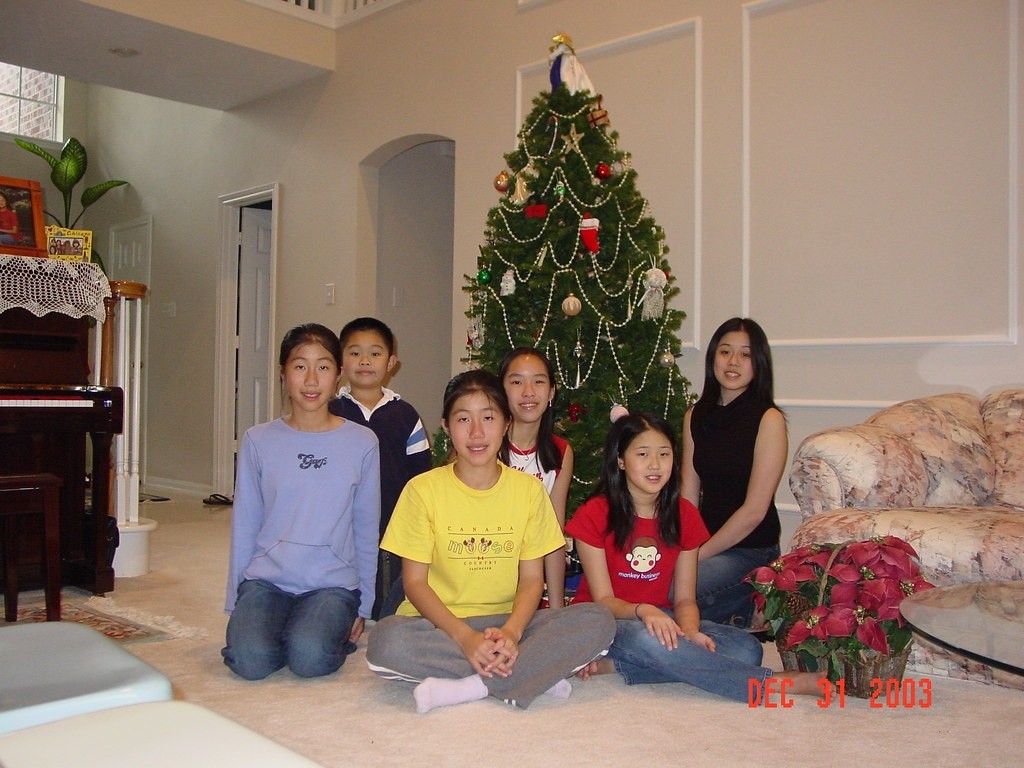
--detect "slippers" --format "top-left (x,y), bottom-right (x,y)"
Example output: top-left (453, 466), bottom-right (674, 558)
top-left (203, 493), bottom-right (234, 505)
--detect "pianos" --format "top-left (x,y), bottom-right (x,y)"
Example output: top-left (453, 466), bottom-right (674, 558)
top-left (0, 254), bottom-right (124, 598)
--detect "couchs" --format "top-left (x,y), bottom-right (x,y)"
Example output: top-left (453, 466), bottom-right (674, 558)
top-left (786, 388), bottom-right (1024, 691)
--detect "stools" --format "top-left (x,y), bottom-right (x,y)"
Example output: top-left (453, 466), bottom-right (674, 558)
top-left (0, 472), bottom-right (64, 625)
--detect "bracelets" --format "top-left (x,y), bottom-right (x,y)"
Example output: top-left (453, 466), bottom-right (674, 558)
top-left (634, 600), bottom-right (643, 622)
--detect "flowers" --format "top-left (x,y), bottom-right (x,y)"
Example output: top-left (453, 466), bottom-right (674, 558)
top-left (740, 536), bottom-right (937, 683)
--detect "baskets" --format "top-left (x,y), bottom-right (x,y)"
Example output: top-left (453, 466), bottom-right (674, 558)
top-left (776, 538), bottom-right (912, 699)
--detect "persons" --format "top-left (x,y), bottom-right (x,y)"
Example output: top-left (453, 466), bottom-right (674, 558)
top-left (222, 323), bottom-right (383, 680)
top-left (666, 318), bottom-right (790, 628)
top-left (561, 410), bottom-right (836, 700)
top-left (364, 370), bottom-right (615, 713)
top-left (446, 347), bottom-right (575, 610)
top-left (319, 318), bottom-right (431, 621)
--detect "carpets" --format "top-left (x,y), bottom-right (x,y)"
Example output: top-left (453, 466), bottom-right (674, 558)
top-left (0, 587), bottom-right (206, 648)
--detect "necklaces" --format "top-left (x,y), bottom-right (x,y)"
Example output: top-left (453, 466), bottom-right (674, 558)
top-left (506, 435), bottom-right (538, 460)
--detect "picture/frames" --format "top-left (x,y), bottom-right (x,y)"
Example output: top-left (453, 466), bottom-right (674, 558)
top-left (45, 224), bottom-right (92, 263)
top-left (0, 176), bottom-right (48, 258)
